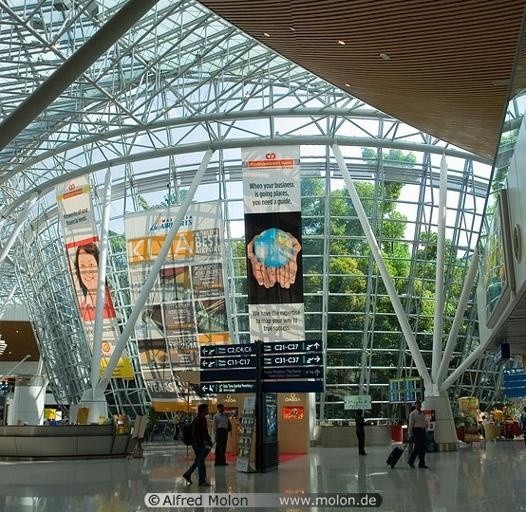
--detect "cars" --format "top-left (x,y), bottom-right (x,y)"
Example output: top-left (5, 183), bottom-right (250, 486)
top-left (364, 417), bottom-right (398, 426)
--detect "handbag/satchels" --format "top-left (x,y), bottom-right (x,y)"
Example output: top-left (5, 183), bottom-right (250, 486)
top-left (227, 419), bottom-right (232, 432)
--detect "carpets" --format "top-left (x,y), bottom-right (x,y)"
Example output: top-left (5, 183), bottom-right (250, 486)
top-left (176, 448), bottom-right (306, 467)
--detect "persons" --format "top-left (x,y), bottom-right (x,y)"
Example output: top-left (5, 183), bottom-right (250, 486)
top-left (212, 404), bottom-right (232, 466)
top-left (472, 415), bottom-right (489, 439)
top-left (355, 408), bottom-right (368, 456)
top-left (406, 400), bottom-right (429, 470)
top-left (172, 410), bottom-right (181, 441)
top-left (183, 403), bottom-right (214, 487)
top-left (521, 405), bottom-right (526, 448)
top-left (73, 242), bottom-right (116, 320)
top-left (406, 406), bottom-right (416, 458)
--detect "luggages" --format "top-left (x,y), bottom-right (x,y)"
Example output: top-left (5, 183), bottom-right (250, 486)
top-left (386, 441), bottom-right (408, 469)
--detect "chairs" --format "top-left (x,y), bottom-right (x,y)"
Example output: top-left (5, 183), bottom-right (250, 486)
top-left (455, 415), bottom-right (525, 448)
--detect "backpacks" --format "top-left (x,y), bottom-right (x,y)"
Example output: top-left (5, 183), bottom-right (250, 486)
top-left (182, 423), bottom-right (193, 447)
